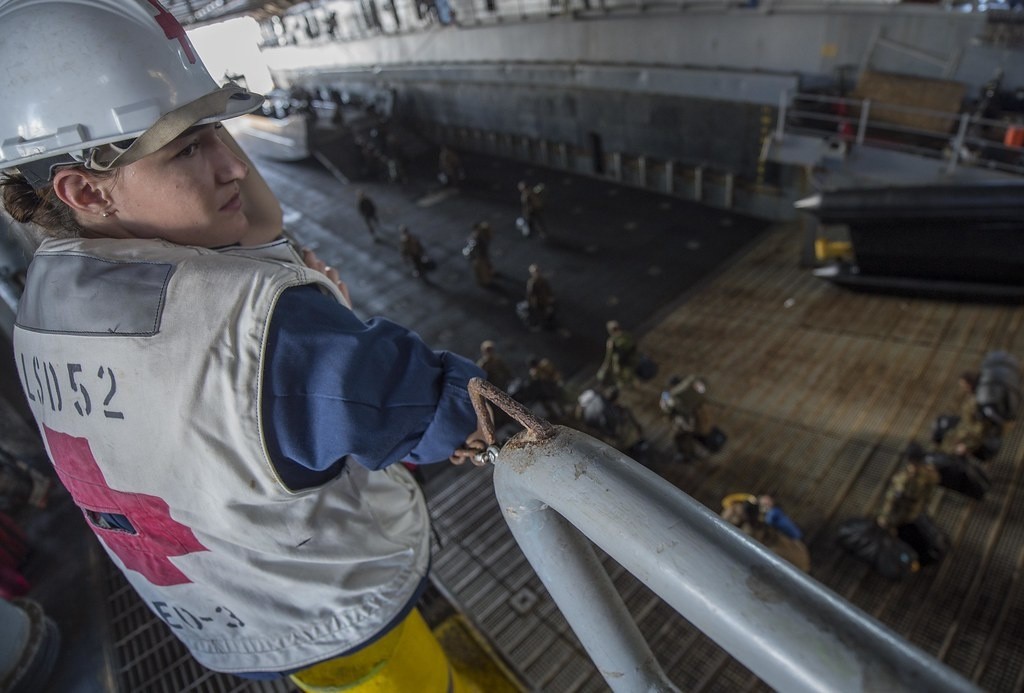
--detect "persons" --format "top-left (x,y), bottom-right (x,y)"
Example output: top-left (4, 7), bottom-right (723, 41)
top-left (596, 321), bottom-right (637, 388)
top-left (527, 356), bottom-right (564, 418)
top-left (0, 0), bottom-right (495, 693)
top-left (400, 224), bottom-right (425, 278)
top-left (465, 220), bottom-right (491, 286)
top-left (882, 440), bottom-right (942, 573)
top-left (722, 496), bottom-right (811, 575)
top-left (440, 143), bottom-right (461, 186)
top-left (945, 371), bottom-right (1002, 469)
top-left (357, 191), bottom-right (381, 242)
top-left (288, 80), bottom-right (405, 183)
top-left (514, 180), bottom-right (544, 237)
top-left (526, 265), bottom-right (571, 337)
top-left (981, 69), bottom-right (1007, 120)
top-left (573, 387), bottom-right (628, 434)
top-left (476, 340), bottom-right (511, 393)
top-left (659, 376), bottom-right (705, 463)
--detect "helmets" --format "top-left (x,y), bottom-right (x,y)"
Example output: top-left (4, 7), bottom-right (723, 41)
top-left (0, 0), bottom-right (266, 169)
